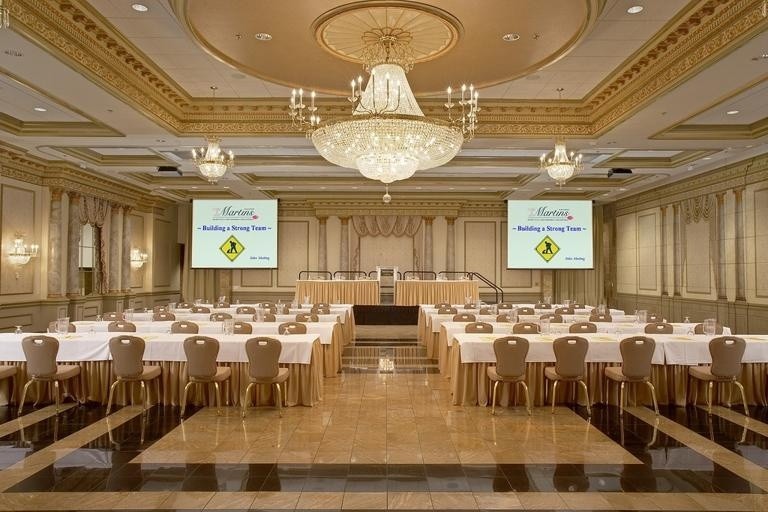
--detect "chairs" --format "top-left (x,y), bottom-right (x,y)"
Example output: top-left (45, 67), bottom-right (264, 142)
top-left (0, 365), bottom-right (18, 403)
top-left (49, 301), bottom-right (331, 335)
top-left (604, 336), bottom-right (660, 417)
top-left (105, 335), bottom-right (162, 414)
top-left (433, 301), bottom-right (724, 335)
top-left (180, 336), bottom-right (232, 417)
top-left (688, 336), bottom-right (750, 417)
top-left (17, 335), bottom-right (82, 416)
top-left (486, 335), bottom-right (532, 417)
top-left (242, 337), bottom-right (290, 419)
top-left (544, 336), bottom-right (593, 417)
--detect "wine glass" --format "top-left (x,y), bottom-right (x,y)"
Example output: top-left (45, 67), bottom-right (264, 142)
top-left (14, 326), bottom-right (23, 337)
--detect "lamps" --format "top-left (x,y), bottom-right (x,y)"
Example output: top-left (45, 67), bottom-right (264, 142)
top-left (286, 1), bottom-right (482, 204)
top-left (538, 86), bottom-right (585, 191)
top-left (8, 234), bottom-right (39, 280)
top-left (128, 247), bottom-right (149, 272)
top-left (156, 165), bottom-right (184, 179)
top-left (606, 166), bottom-right (634, 182)
top-left (191, 86), bottom-right (236, 186)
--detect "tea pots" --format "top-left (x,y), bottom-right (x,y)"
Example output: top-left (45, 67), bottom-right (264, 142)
top-left (464, 296), bottom-right (716, 338)
top-left (58, 295), bottom-right (310, 337)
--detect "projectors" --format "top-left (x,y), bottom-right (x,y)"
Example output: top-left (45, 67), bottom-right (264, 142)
top-left (158, 167), bottom-right (182, 177)
top-left (608, 168), bottom-right (633, 179)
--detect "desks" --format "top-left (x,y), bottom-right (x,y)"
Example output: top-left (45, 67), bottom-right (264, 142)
top-left (294, 278), bottom-right (383, 307)
top-left (393, 277), bottom-right (480, 308)
